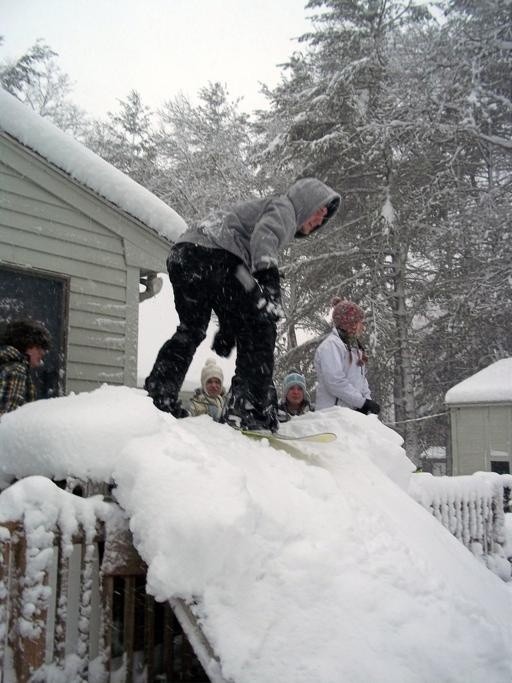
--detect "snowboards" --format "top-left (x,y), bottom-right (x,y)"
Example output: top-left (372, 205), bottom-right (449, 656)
top-left (239, 427), bottom-right (337, 443)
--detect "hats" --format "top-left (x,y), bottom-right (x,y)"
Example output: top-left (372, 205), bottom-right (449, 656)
top-left (202, 358), bottom-right (223, 396)
top-left (3, 320), bottom-right (50, 348)
top-left (332, 297), bottom-right (364, 335)
top-left (283, 372), bottom-right (307, 401)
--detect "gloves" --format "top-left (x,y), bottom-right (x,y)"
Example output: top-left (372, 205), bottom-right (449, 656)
top-left (212, 318), bottom-right (234, 357)
top-left (357, 399), bottom-right (380, 415)
top-left (259, 270), bottom-right (281, 321)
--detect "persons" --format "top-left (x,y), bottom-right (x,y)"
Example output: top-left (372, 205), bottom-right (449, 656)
top-left (280, 372), bottom-right (315, 423)
top-left (313, 295), bottom-right (381, 417)
top-left (184, 356), bottom-right (227, 422)
top-left (0, 318), bottom-right (53, 415)
top-left (135, 176), bottom-right (343, 429)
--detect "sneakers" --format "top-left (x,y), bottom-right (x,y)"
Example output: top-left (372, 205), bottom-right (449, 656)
top-left (223, 410), bottom-right (279, 433)
top-left (144, 377), bottom-right (188, 418)
